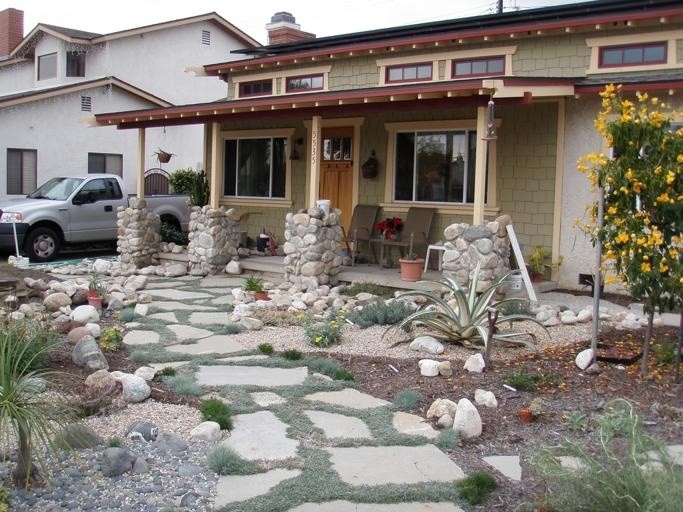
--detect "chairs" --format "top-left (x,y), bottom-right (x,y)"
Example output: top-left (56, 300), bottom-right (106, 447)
top-left (384, 205), bottom-right (437, 270)
top-left (335, 203), bottom-right (380, 268)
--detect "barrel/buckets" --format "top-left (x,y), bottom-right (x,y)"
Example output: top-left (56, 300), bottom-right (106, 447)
top-left (317, 199), bottom-right (330, 217)
top-left (511, 274), bottom-right (524, 290)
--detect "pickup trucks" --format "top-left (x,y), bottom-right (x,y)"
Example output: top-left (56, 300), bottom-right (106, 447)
top-left (0, 173), bottom-right (198, 262)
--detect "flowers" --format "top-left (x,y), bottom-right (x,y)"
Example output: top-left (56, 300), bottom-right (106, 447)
top-left (374, 216), bottom-right (403, 230)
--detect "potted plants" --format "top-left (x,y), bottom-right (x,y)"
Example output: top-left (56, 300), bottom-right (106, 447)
top-left (244, 275), bottom-right (265, 302)
top-left (398, 247), bottom-right (425, 282)
top-left (85, 279), bottom-right (106, 310)
top-left (151, 147), bottom-right (176, 164)
top-left (529, 242), bottom-right (546, 282)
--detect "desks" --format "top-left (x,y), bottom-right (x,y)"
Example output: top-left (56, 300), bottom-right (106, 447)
top-left (368, 239), bottom-right (388, 264)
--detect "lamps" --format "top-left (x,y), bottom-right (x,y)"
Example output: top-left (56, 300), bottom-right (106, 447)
top-left (289, 137), bottom-right (304, 161)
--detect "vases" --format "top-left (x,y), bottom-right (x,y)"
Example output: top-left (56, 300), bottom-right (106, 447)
top-left (255, 291), bottom-right (268, 301)
top-left (385, 229), bottom-right (395, 240)
